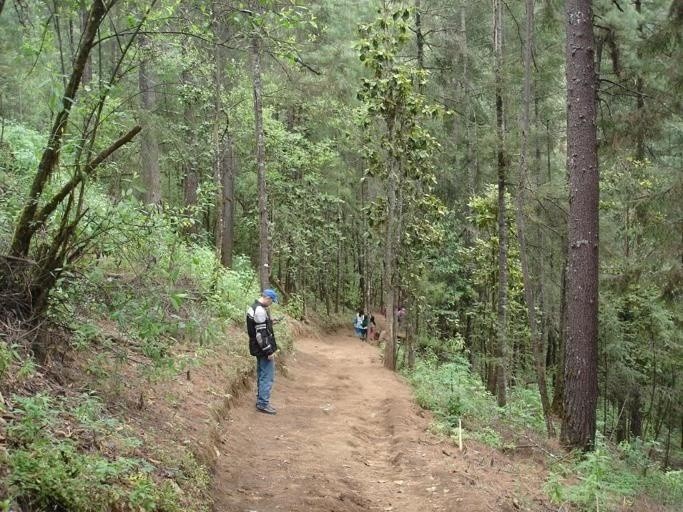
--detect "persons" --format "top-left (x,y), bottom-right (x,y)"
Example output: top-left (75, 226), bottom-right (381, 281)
top-left (352, 309), bottom-right (364, 335)
top-left (246, 288), bottom-right (287, 415)
top-left (359, 314), bottom-right (368, 340)
top-left (363, 316), bottom-right (376, 344)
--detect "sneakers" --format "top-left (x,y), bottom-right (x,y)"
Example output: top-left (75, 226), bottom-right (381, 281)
top-left (256, 402), bottom-right (276, 413)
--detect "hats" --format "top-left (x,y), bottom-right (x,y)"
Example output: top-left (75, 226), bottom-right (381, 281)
top-left (263, 290), bottom-right (279, 304)
